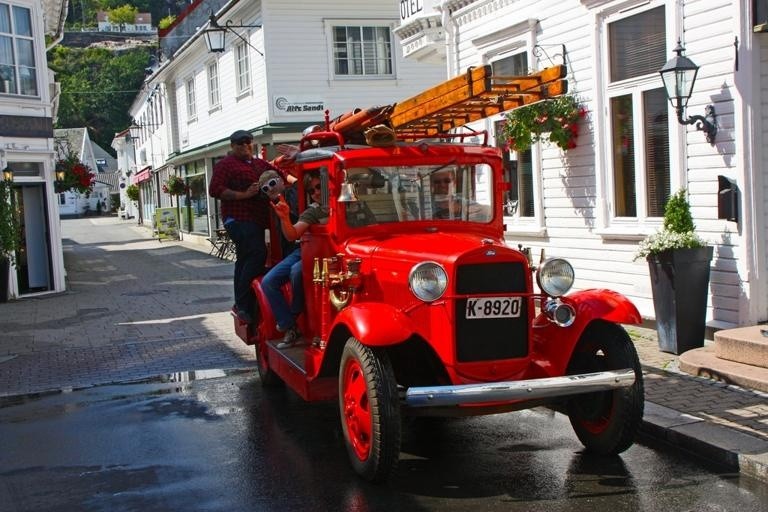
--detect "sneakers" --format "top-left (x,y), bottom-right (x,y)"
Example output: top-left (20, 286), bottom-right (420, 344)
top-left (276, 323), bottom-right (306, 350)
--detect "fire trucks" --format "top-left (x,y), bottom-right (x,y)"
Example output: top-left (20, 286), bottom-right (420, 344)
top-left (230, 64), bottom-right (645, 479)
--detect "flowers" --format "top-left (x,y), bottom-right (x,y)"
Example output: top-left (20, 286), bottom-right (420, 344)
top-left (161, 175), bottom-right (189, 196)
top-left (54, 137), bottom-right (98, 196)
top-left (494, 95), bottom-right (589, 153)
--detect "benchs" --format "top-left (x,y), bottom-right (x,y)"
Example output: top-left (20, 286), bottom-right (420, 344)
top-left (206, 237), bottom-right (237, 263)
top-left (358, 192), bottom-right (436, 222)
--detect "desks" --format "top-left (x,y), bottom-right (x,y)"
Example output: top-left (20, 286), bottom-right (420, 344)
top-left (213, 229), bottom-right (236, 259)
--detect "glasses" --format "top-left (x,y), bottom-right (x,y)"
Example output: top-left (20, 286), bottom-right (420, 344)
top-left (233, 139), bottom-right (252, 146)
top-left (306, 183), bottom-right (320, 195)
top-left (261, 177), bottom-right (283, 194)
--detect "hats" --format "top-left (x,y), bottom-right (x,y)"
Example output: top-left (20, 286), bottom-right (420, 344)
top-left (230, 130), bottom-right (253, 140)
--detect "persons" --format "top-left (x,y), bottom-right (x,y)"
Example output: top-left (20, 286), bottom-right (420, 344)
top-left (100, 197), bottom-right (107, 216)
top-left (398, 165), bottom-right (492, 223)
top-left (259, 171), bottom-right (377, 350)
top-left (208, 130), bottom-right (298, 324)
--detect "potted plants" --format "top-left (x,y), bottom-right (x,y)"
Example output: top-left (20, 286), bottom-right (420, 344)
top-left (126, 185), bottom-right (139, 200)
top-left (632, 188), bottom-right (714, 358)
top-left (0, 178), bottom-right (26, 303)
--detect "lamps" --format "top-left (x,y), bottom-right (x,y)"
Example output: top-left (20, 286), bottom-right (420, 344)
top-left (659, 36), bottom-right (717, 146)
top-left (201, 9), bottom-right (264, 56)
top-left (2, 161), bottom-right (14, 186)
top-left (55, 163), bottom-right (66, 181)
